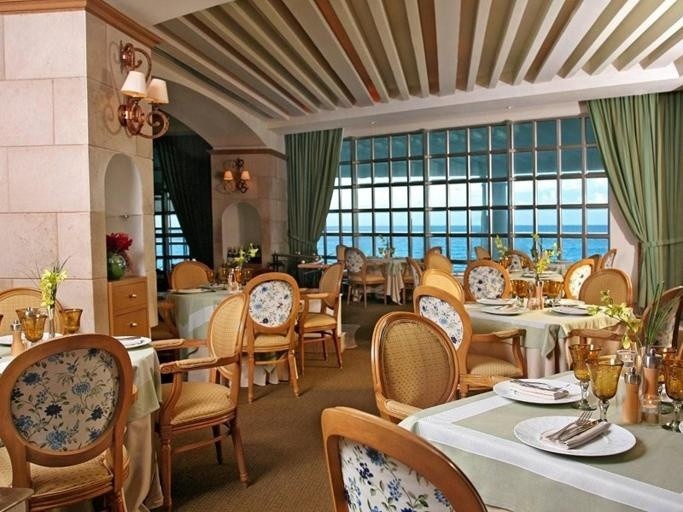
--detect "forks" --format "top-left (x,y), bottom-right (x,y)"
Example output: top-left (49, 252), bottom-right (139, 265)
top-left (545, 409), bottom-right (593, 440)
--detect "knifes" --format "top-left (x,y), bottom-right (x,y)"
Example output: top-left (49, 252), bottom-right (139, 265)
top-left (558, 418), bottom-right (606, 442)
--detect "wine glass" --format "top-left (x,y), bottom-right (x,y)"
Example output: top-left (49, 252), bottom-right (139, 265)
top-left (585, 357), bottom-right (623, 423)
top-left (661, 357), bottom-right (683, 433)
top-left (568, 343), bottom-right (595, 411)
top-left (653, 347), bottom-right (677, 394)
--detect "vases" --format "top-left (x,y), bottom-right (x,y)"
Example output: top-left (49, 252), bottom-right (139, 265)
top-left (46, 307), bottom-right (57, 338)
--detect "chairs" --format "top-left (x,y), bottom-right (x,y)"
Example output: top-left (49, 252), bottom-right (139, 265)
top-left (474, 246), bottom-right (491, 261)
top-left (336, 245), bottom-right (346, 266)
top-left (2, 289), bottom-right (65, 338)
top-left (2, 334), bottom-right (137, 510)
top-left (579, 268), bottom-right (632, 312)
top-left (240, 271), bottom-right (301, 401)
top-left (463, 260), bottom-right (511, 301)
top-left (343, 248), bottom-right (387, 307)
top-left (421, 269), bottom-right (465, 307)
top-left (171, 260), bottom-right (214, 293)
top-left (296, 260), bottom-right (345, 372)
top-left (412, 287), bottom-right (527, 400)
top-left (601, 248), bottom-right (617, 270)
top-left (588, 254), bottom-right (600, 271)
top-left (406, 257), bottom-right (423, 285)
top-left (147, 291), bottom-right (251, 508)
top-left (501, 251), bottom-right (533, 273)
top-left (570, 285), bottom-right (682, 368)
top-left (425, 251), bottom-right (454, 275)
top-left (564, 260), bottom-right (596, 299)
top-left (403, 247), bottom-right (442, 306)
top-left (370, 311), bottom-right (460, 426)
top-left (321, 405), bottom-right (487, 509)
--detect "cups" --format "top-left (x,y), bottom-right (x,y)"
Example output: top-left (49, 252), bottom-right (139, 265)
top-left (640, 394), bottom-right (662, 427)
top-left (617, 350), bottom-right (637, 377)
top-left (21, 314), bottom-right (47, 346)
top-left (15, 307), bottom-right (40, 323)
top-left (58, 308), bottom-right (82, 334)
top-left (205, 264), bottom-right (252, 290)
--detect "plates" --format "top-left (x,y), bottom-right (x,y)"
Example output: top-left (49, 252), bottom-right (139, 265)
top-left (552, 306), bottom-right (590, 315)
top-left (546, 298), bottom-right (585, 306)
top-left (480, 306), bottom-right (529, 315)
top-left (168, 287), bottom-right (202, 294)
top-left (0, 335), bottom-right (25, 345)
top-left (512, 416), bottom-right (637, 457)
top-left (114, 336), bottom-right (151, 350)
top-left (492, 379), bottom-right (584, 404)
top-left (474, 298), bottom-right (514, 305)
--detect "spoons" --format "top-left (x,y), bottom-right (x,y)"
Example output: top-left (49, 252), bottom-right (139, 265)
top-left (515, 378), bottom-right (570, 390)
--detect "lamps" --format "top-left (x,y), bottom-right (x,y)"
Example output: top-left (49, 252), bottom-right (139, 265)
top-left (223, 158), bottom-right (250, 193)
top-left (117, 40), bottom-right (170, 139)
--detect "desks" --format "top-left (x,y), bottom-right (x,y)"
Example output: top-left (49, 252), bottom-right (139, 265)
top-left (461, 298), bottom-right (634, 379)
top-left (395, 366), bottom-right (682, 510)
top-left (365, 256), bottom-right (407, 306)
top-left (1, 331), bottom-right (165, 510)
top-left (156, 289), bottom-right (289, 389)
top-left (453, 267), bottom-right (565, 299)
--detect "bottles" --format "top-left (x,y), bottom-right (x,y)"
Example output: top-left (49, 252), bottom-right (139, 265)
top-left (10, 319), bottom-right (23, 357)
top-left (623, 367), bottom-right (642, 424)
top-left (641, 348), bottom-right (659, 399)
top-left (226, 240), bottom-right (259, 264)
top-left (526, 280), bottom-right (543, 309)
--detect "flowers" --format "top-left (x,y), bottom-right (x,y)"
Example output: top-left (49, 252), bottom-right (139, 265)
top-left (225, 246), bottom-right (259, 268)
top-left (16, 250), bottom-right (74, 308)
top-left (106, 232), bottom-right (133, 253)
top-left (524, 245), bottom-right (559, 275)
top-left (583, 283), bottom-right (676, 353)
top-left (377, 235), bottom-right (394, 255)
